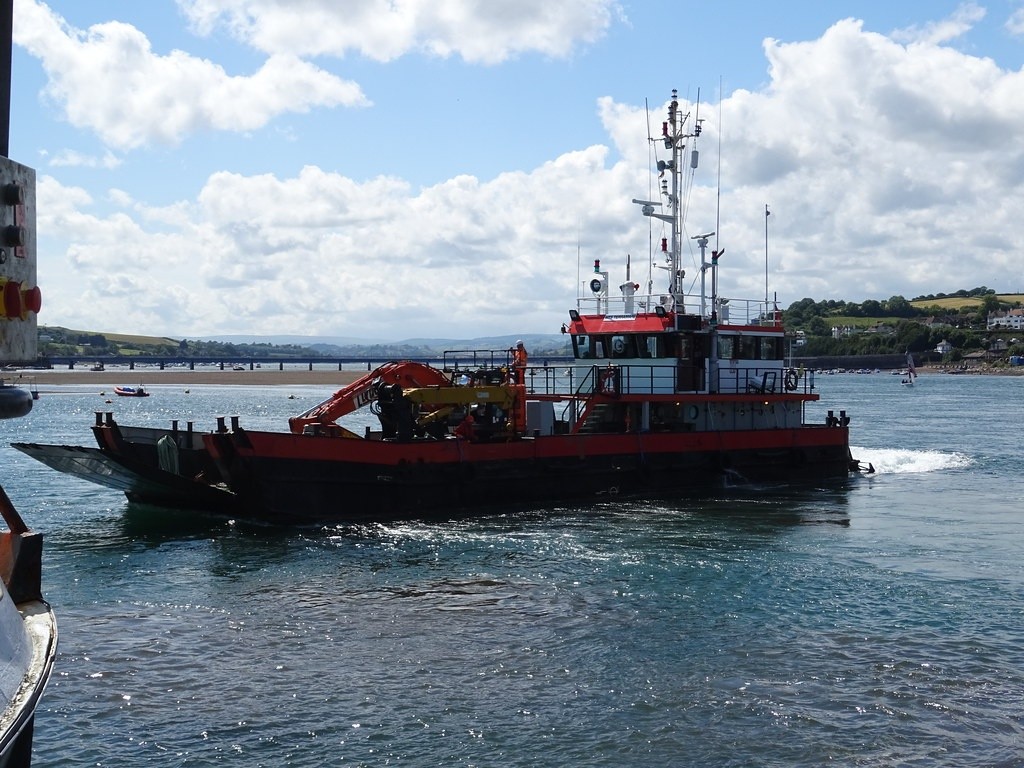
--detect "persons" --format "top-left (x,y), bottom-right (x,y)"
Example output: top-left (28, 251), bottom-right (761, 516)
top-left (505, 340), bottom-right (528, 385)
top-left (453, 415), bottom-right (479, 440)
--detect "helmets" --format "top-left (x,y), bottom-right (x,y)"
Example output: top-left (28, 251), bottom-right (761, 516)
top-left (516, 340), bottom-right (523, 345)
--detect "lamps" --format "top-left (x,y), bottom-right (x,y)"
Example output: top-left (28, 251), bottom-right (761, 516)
top-left (569, 309), bottom-right (582, 323)
top-left (656, 306), bottom-right (670, 320)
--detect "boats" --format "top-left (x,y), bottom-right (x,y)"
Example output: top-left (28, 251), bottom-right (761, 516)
top-left (113, 383), bottom-right (150, 397)
top-left (8, 80), bottom-right (875, 535)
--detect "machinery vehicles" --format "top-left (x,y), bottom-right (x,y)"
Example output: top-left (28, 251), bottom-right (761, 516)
top-left (287, 358), bottom-right (523, 445)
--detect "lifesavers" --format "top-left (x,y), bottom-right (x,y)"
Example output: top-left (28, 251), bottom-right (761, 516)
top-left (785, 371), bottom-right (798, 391)
top-left (600, 373), bottom-right (616, 396)
